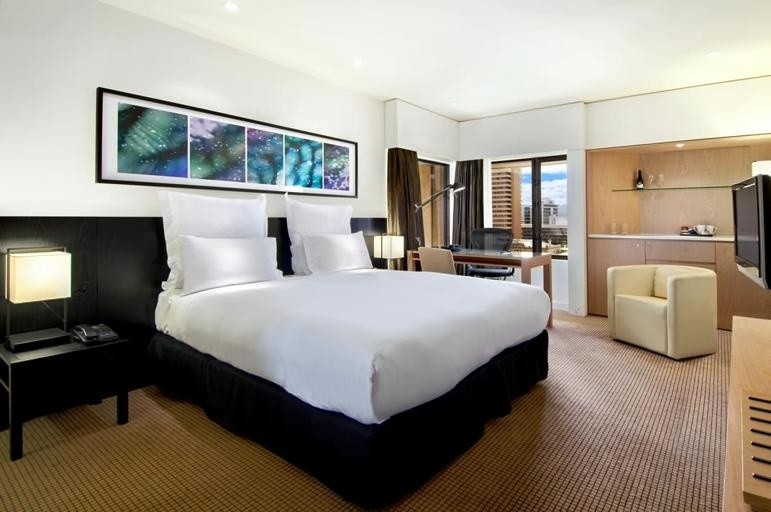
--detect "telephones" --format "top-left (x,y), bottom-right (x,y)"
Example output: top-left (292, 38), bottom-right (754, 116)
top-left (72, 322), bottom-right (120, 347)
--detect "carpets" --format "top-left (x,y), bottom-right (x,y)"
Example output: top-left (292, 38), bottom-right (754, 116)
top-left (1, 321), bottom-right (732, 511)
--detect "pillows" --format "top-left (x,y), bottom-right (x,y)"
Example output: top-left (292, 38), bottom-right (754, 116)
top-left (301, 230), bottom-right (374, 274)
top-left (178, 235), bottom-right (284, 297)
top-left (284, 192), bottom-right (353, 276)
top-left (158, 190), bottom-right (267, 290)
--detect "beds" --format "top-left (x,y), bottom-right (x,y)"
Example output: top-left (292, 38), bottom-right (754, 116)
top-left (157, 190), bottom-right (551, 512)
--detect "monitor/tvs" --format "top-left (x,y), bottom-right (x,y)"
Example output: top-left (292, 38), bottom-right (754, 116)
top-left (731, 175), bottom-right (771, 291)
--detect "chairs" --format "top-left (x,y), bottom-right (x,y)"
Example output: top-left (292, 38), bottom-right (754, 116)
top-left (418, 246), bottom-right (457, 275)
top-left (468, 228), bottom-right (515, 280)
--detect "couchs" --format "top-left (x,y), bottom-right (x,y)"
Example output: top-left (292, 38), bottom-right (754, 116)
top-left (607, 264), bottom-right (717, 359)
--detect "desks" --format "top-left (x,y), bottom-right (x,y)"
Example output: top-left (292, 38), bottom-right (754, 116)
top-left (407, 252), bottom-right (552, 327)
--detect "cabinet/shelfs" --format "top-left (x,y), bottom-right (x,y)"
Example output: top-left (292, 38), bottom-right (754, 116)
top-left (585, 134), bottom-right (771, 330)
top-left (724, 315), bottom-right (771, 512)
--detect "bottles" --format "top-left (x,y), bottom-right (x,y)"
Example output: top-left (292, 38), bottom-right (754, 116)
top-left (636, 170), bottom-right (643, 190)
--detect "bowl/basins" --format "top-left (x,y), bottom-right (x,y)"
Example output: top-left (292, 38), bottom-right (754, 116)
top-left (693, 224), bottom-right (720, 235)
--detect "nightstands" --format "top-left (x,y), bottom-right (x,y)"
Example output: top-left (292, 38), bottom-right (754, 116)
top-left (0, 326), bottom-right (132, 461)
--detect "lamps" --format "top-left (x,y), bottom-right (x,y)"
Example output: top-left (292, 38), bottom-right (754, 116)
top-left (373, 233), bottom-right (404, 269)
top-left (4, 247), bottom-right (73, 354)
top-left (414, 182), bottom-right (465, 250)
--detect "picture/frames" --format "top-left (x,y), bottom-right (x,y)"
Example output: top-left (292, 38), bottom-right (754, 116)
top-left (95, 86), bottom-right (358, 198)
